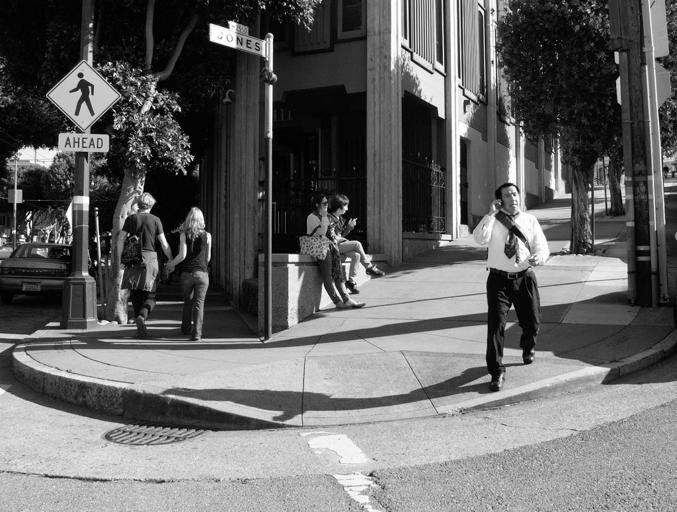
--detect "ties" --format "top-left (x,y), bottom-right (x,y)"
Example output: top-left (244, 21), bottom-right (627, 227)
top-left (504, 216), bottom-right (520, 264)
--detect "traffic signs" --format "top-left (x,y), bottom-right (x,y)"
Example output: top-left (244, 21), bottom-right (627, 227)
top-left (208, 23), bottom-right (267, 61)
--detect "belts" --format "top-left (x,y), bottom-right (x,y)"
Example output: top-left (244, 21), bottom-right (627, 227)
top-left (489, 267), bottom-right (532, 280)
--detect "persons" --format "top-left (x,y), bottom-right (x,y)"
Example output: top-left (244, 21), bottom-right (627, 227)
top-left (471, 182), bottom-right (551, 395)
top-left (68, 71), bottom-right (95, 118)
top-left (328, 192), bottom-right (385, 296)
top-left (160, 207), bottom-right (212, 342)
top-left (118, 192), bottom-right (176, 342)
top-left (305, 193), bottom-right (366, 313)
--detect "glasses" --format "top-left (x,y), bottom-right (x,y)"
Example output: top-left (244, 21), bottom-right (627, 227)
top-left (320, 201), bottom-right (328, 206)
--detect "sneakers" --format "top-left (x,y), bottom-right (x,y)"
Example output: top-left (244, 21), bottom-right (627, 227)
top-left (366, 264), bottom-right (386, 277)
top-left (135, 316), bottom-right (152, 338)
top-left (345, 280), bottom-right (360, 294)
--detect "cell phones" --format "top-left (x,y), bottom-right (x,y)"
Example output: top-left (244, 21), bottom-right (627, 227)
top-left (497, 201), bottom-right (503, 207)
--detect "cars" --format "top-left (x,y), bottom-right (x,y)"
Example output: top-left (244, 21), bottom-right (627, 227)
top-left (0, 241), bottom-right (96, 306)
top-left (0, 232), bottom-right (113, 275)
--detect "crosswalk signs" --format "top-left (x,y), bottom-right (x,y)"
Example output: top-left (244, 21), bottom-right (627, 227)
top-left (43, 59), bottom-right (122, 131)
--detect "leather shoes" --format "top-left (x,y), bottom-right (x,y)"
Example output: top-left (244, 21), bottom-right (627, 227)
top-left (523, 347), bottom-right (536, 364)
top-left (489, 372), bottom-right (504, 391)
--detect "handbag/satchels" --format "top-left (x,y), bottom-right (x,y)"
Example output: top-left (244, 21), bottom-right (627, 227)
top-left (298, 234), bottom-right (330, 261)
top-left (121, 232), bottom-right (145, 267)
